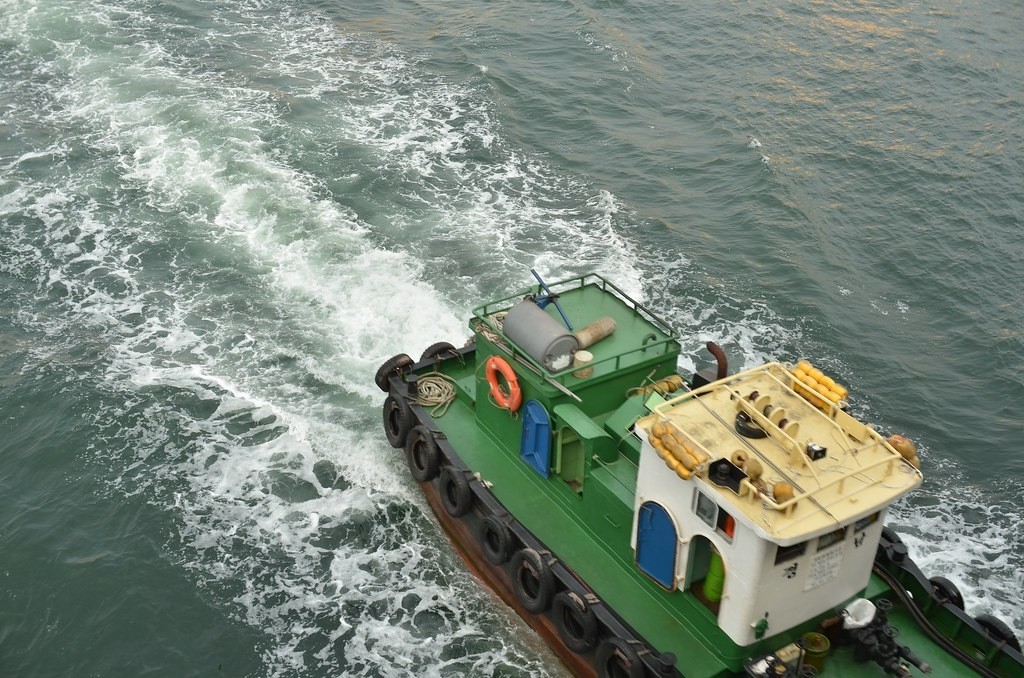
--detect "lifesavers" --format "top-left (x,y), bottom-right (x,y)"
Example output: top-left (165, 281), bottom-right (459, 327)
top-left (437, 465), bottom-right (471, 518)
top-left (419, 342), bottom-right (456, 362)
top-left (594, 637), bottom-right (645, 678)
top-left (928, 575), bottom-right (964, 612)
top-left (375, 353), bottom-right (416, 393)
top-left (485, 353), bottom-right (523, 414)
top-left (735, 410), bottom-right (770, 439)
top-left (974, 612), bottom-right (1022, 653)
top-left (882, 522), bottom-right (907, 551)
top-left (552, 590), bottom-right (598, 653)
top-left (383, 394), bottom-right (411, 449)
top-left (405, 425), bottom-right (440, 484)
top-left (479, 514), bottom-right (512, 565)
top-left (511, 547), bottom-right (556, 614)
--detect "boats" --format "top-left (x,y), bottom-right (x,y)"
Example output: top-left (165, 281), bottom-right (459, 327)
top-left (377, 271), bottom-right (1024, 678)
